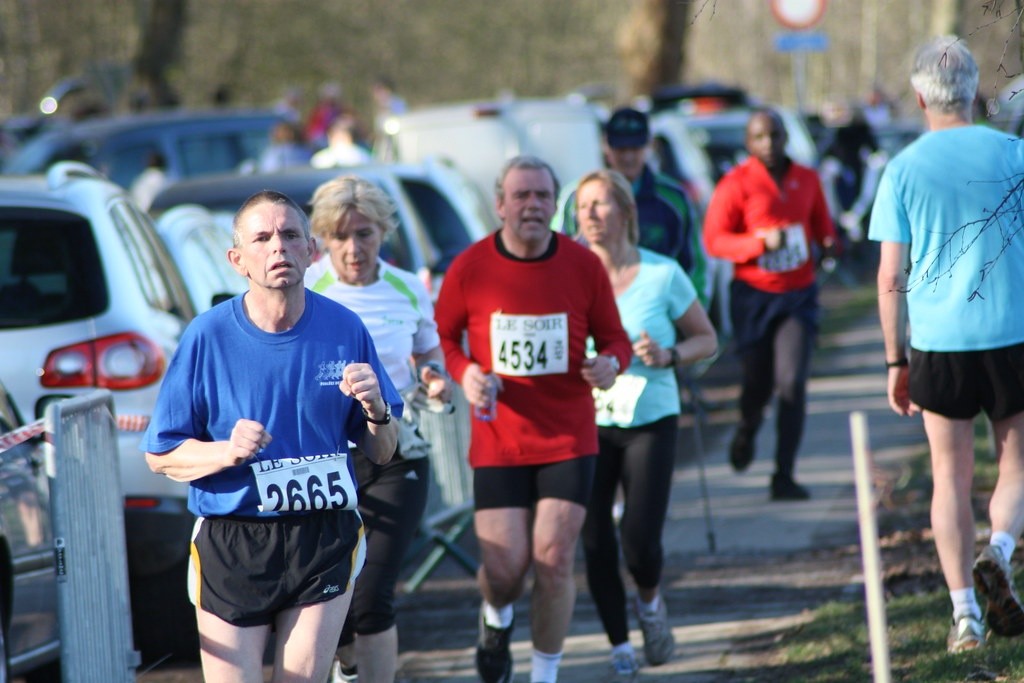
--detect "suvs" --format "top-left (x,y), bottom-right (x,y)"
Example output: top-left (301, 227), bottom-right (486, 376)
top-left (0, 84), bottom-right (819, 577)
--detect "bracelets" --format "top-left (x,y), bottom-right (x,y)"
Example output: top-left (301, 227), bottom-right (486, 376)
top-left (885, 358), bottom-right (910, 369)
top-left (666, 348), bottom-right (680, 368)
top-left (415, 360), bottom-right (450, 389)
top-left (606, 354), bottom-right (621, 373)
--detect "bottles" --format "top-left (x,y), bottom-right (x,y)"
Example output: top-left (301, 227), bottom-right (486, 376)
top-left (473, 364), bottom-right (498, 420)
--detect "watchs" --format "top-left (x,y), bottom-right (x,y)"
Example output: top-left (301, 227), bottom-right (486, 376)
top-left (360, 400), bottom-right (392, 424)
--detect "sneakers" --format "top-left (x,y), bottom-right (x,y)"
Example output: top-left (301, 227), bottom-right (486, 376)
top-left (475, 602), bottom-right (515, 683)
top-left (635, 594), bottom-right (673, 667)
top-left (972, 546), bottom-right (1024, 638)
top-left (946, 613), bottom-right (985, 656)
top-left (611, 657), bottom-right (641, 683)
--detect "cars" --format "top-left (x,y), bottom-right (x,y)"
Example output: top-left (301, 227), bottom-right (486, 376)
top-left (0, 382), bottom-right (58, 683)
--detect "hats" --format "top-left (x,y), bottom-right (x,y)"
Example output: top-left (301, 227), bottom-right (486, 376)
top-left (606, 107), bottom-right (648, 148)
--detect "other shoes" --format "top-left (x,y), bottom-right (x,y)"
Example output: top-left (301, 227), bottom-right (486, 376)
top-left (773, 478), bottom-right (808, 501)
top-left (730, 421), bottom-right (753, 472)
top-left (332, 660), bottom-right (359, 683)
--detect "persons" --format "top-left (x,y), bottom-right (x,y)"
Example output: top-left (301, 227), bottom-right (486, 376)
top-left (566, 168), bottom-right (717, 682)
top-left (867, 34), bottom-right (1024, 656)
top-left (863, 84), bottom-right (899, 126)
top-left (139, 190), bottom-right (404, 683)
top-left (237, 72), bottom-right (410, 179)
top-left (703, 110), bottom-right (838, 501)
top-left (550, 107), bottom-right (705, 391)
top-left (302, 175), bottom-right (452, 683)
top-left (125, 150), bottom-right (171, 211)
top-left (433, 156), bottom-right (633, 682)
